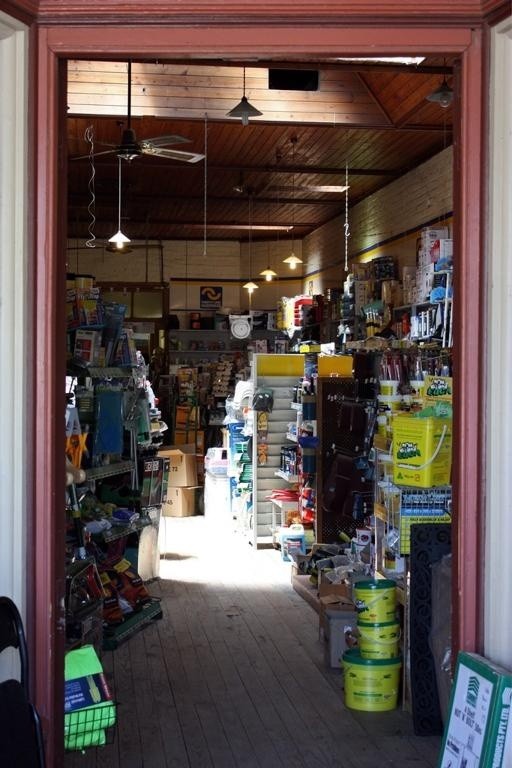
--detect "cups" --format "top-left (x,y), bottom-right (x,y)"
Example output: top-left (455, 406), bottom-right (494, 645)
top-left (409, 381), bottom-right (424, 395)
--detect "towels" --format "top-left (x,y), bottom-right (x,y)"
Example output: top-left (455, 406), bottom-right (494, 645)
top-left (64, 644), bottom-right (115, 750)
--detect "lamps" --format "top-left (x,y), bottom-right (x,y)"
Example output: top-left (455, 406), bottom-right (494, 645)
top-left (281, 136), bottom-right (304, 269)
top-left (425, 55), bottom-right (453, 107)
top-left (258, 162), bottom-right (277, 280)
top-left (226, 68), bottom-right (263, 126)
top-left (243, 188), bottom-right (258, 293)
top-left (108, 154), bottom-right (132, 249)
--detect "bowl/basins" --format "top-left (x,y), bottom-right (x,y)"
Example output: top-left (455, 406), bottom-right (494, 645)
top-left (352, 527), bottom-right (372, 558)
top-left (378, 380), bottom-right (401, 435)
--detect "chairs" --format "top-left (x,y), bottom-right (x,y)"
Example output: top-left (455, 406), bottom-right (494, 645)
top-left (0, 595), bottom-right (45, 767)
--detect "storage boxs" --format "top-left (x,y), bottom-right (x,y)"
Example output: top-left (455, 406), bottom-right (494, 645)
top-left (154, 429), bottom-right (204, 516)
top-left (176, 405), bottom-right (201, 429)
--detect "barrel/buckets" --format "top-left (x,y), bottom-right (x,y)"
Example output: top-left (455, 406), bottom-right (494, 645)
top-left (282, 523), bottom-right (307, 564)
top-left (343, 661), bottom-right (400, 713)
top-left (355, 624), bottom-right (401, 659)
top-left (351, 580), bottom-right (394, 624)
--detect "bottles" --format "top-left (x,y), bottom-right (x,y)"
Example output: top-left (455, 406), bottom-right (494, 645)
top-left (280, 446), bottom-right (299, 475)
top-left (214, 312), bottom-right (227, 330)
top-left (71, 302), bottom-right (87, 326)
top-left (191, 312), bottom-right (201, 329)
top-left (292, 385), bottom-right (301, 403)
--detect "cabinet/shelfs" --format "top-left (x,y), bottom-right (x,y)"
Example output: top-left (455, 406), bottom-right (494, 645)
top-left (265, 402), bottom-right (302, 547)
top-left (300, 299), bottom-right (449, 349)
top-left (63, 372), bottom-right (165, 648)
top-left (168, 329), bottom-right (244, 365)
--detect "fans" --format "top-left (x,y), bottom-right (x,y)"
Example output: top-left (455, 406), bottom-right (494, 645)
top-left (64, 62), bottom-right (206, 164)
top-left (68, 193), bottom-right (164, 253)
